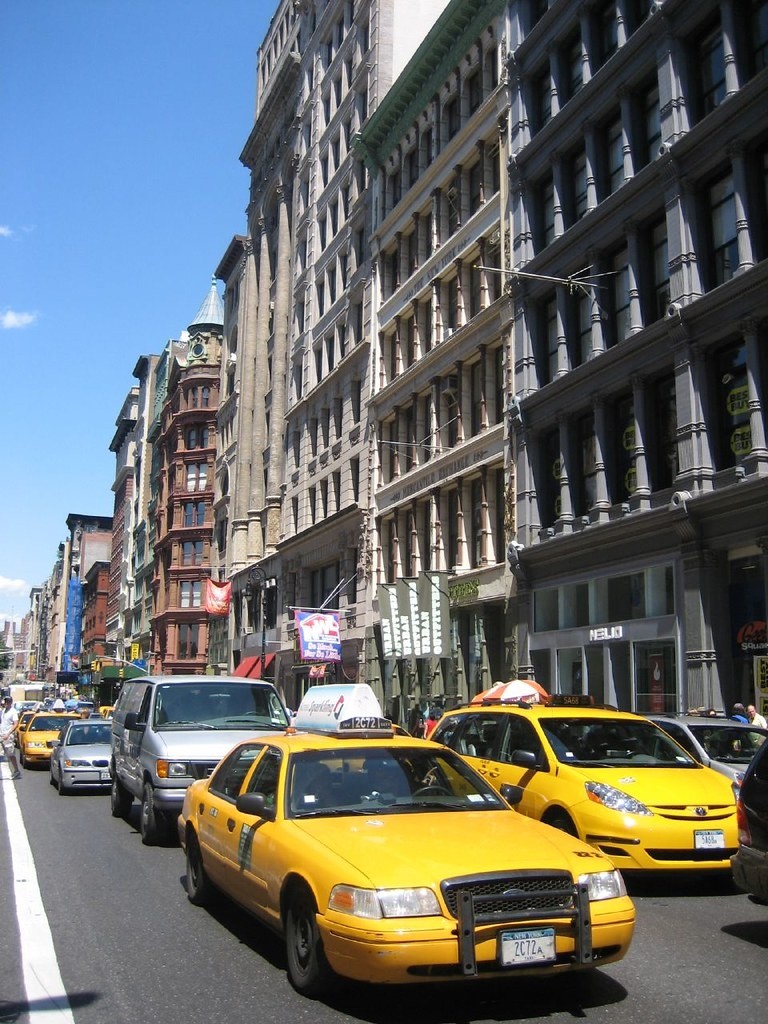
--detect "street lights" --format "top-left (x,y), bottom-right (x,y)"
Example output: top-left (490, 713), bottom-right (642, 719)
top-left (245, 567), bottom-right (267, 681)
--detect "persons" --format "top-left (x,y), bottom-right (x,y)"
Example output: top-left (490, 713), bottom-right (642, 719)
top-left (0, 696), bottom-right (22, 780)
top-left (297, 753), bottom-right (437, 809)
top-left (686, 703), bottom-right (768, 751)
top-left (416, 710), bottom-right (443, 739)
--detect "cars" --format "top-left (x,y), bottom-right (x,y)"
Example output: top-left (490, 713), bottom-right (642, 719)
top-left (0, 685), bottom-right (117, 751)
top-left (19, 699), bottom-right (87, 771)
top-left (49, 717), bottom-right (114, 796)
top-left (177, 683), bottom-right (637, 1001)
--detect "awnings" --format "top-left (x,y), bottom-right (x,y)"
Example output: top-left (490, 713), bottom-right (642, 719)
top-left (232, 652), bottom-right (275, 679)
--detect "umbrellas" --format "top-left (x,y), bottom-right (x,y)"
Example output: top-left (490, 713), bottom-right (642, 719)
top-left (470, 679), bottom-right (549, 707)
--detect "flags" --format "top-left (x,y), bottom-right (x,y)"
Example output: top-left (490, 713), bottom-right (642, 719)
top-left (205, 577), bottom-right (232, 617)
top-left (293, 610), bottom-right (342, 662)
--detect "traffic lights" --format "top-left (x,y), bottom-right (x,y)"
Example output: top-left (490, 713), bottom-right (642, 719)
top-left (119, 668), bottom-right (125, 679)
top-left (91, 660), bottom-right (97, 671)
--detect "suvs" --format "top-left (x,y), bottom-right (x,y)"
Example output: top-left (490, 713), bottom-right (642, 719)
top-left (730, 736), bottom-right (768, 904)
top-left (109, 674), bottom-right (307, 848)
top-left (420, 694), bottom-right (738, 878)
top-left (617, 708), bottom-right (768, 790)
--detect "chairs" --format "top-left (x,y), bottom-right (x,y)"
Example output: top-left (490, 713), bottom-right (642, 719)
top-left (585, 729), bottom-right (627, 760)
